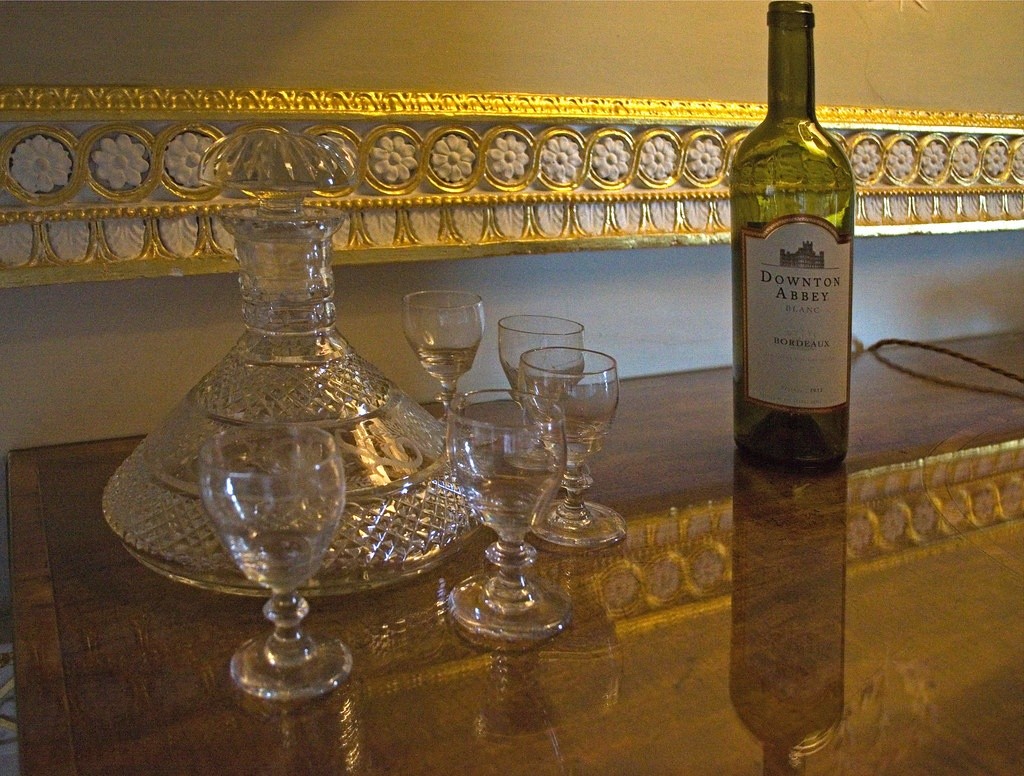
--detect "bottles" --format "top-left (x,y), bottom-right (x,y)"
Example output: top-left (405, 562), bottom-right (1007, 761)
top-left (729, 0), bottom-right (856, 475)
top-left (101, 133), bottom-right (473, 599)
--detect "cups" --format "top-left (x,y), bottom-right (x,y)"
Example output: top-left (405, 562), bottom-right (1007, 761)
top-left (497, 314), bottom-right (586, 405)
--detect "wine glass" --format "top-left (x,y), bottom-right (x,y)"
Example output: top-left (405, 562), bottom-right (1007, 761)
top-left (514, 346), bottom-right (628, 549)
top-left (199, 423), bottom-right (353, 702)
top-left (401, 291), bottom-right (486, 426)
top-left (449, 388), bottom-right (574, 642)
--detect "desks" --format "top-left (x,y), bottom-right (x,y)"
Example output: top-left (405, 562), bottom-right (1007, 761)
top-left (8, 331), bottom-right (1024, 776)
